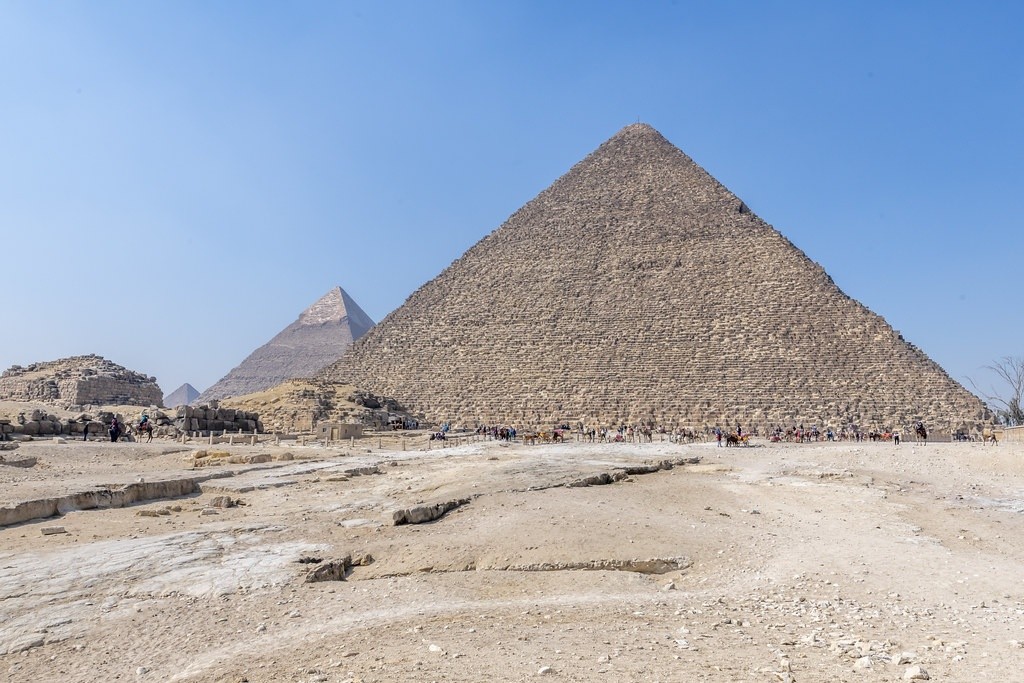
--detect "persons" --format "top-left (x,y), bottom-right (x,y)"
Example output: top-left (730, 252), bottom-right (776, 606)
top-left (768, 423), bottom-right (894, 443)
top-left (83, 421), bottom-right (90, 441)
top-left (475, 422), bottom-right (516, 443)
top-left (138, 414), bottom-right (149, 428)
top-left (735, 425), bottom-right (742, 437)
top-left (108, 418), bottom-right (121, 438)
top-left (399, 416), bottom-right (452, 441)
top-left (894, 430), bottom-right (900, 446)
top-left (918, 421), bottom-right (924, 432)
top-left (715, 427), bottom-right (723, 447)
top-left (552, 420), bottom-right (710, 445)
